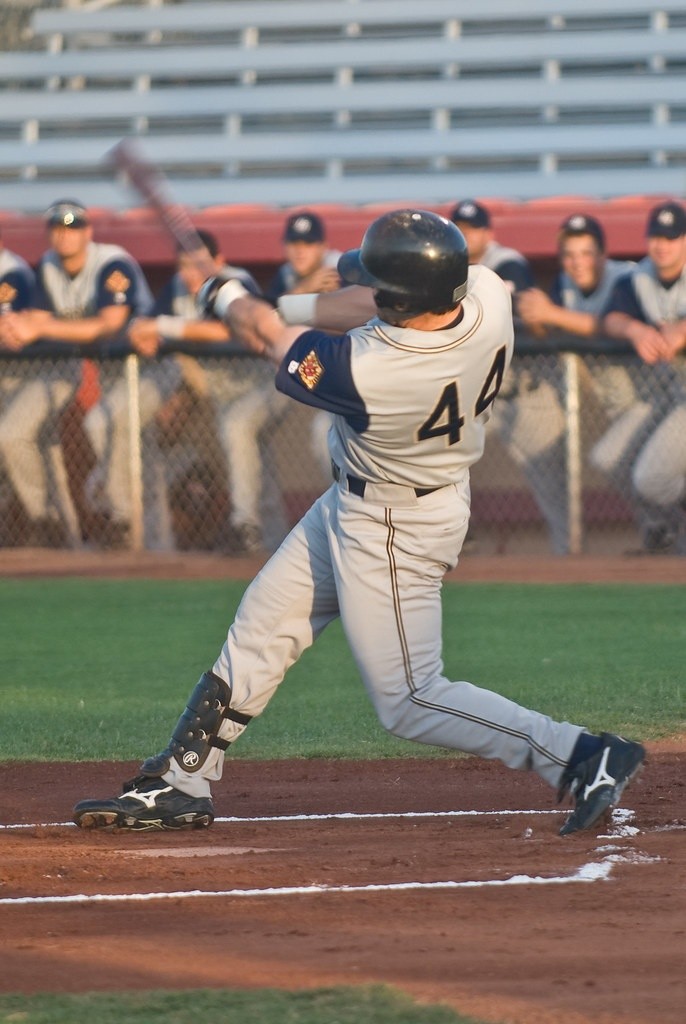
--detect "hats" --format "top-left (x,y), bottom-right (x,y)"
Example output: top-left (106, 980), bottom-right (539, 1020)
top-left (47, 200), bottom-right (88, 228)
top-left (451, 200), bottom-right (489, 228)
top-left (644, 202), bottom-right (686, 239)
top-left (283, 213), bottom-right (323, 241)
top-left (557, 213), bottom-right (604, 254)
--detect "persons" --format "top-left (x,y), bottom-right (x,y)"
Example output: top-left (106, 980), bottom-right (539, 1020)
top-left (70, 207), bottom-right (649, 839)
top-left (0, 201), bottom-right (685, 551)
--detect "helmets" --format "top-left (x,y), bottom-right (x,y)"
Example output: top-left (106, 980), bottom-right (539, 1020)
top-left (337, 207), bottom-right (468, 300)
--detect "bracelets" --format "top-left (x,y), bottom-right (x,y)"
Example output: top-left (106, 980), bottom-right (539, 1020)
top-left (213, 278), bottom-right (247, 318)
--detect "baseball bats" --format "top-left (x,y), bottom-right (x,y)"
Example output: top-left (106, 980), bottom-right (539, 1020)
top-left (110, 135), bottom-right (210, 264)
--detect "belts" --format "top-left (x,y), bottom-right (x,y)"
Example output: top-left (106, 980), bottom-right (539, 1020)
top-left (330, 458), bottom-right (444, 498)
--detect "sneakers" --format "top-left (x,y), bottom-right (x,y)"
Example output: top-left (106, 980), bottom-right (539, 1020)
top-left (554, 730), bottom-right (645, 835)
top-left (72, 775), bottom-right (215, 833)
